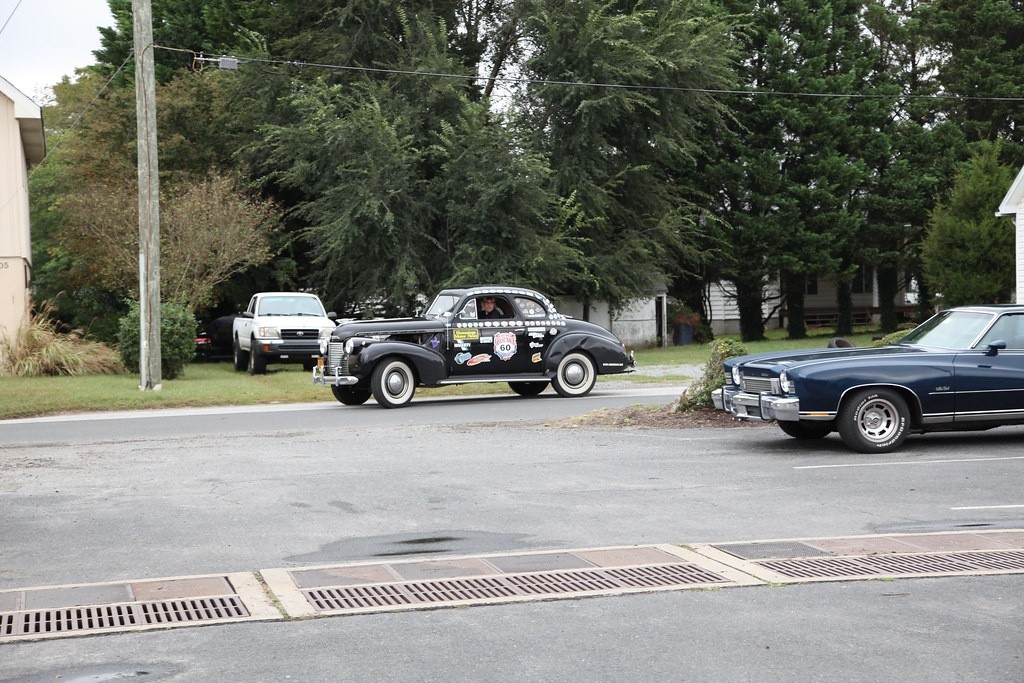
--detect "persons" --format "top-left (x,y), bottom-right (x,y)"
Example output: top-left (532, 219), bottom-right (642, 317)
top-left (478, 296), bottom-right (503, 318)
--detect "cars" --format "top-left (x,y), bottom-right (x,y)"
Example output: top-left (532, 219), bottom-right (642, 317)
top-left (709, 303), bottom-right (1024, 453)
top-left (312, 285), bottom-right (637, 409)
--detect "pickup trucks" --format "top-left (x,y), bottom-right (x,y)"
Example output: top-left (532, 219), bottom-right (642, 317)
top-left (191, 291), bottom-right (338, 376)
